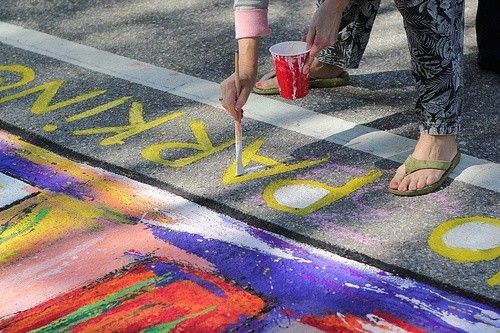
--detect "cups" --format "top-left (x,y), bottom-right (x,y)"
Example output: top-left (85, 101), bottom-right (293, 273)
top-left (270, 40), bottom-right (312, 100)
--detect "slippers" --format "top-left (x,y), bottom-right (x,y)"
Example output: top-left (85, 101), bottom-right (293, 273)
top-left (387, 147), bottom-right (461, 196)
top-left (251, 72), bottom-right (349, 94)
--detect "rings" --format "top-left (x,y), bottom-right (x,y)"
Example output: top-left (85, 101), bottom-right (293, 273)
top-left (219, 97), bottom-right (224, 101)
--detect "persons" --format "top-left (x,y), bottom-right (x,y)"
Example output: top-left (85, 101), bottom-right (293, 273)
top-left (219, 0), bottom-right (466, 197)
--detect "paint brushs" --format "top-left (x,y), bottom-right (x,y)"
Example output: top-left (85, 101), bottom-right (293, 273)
top-left (231, 50), bottom-right (246, 176)
